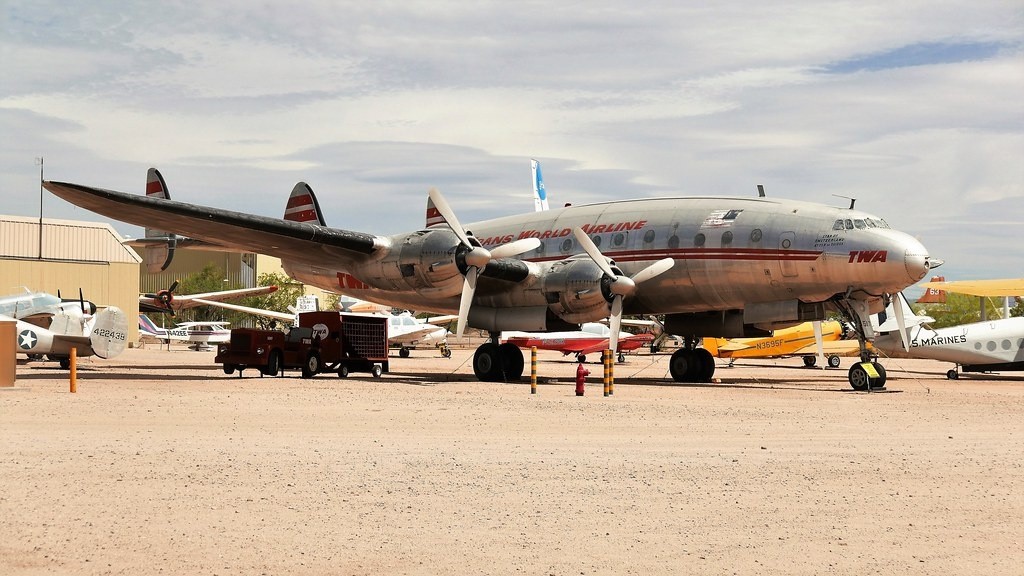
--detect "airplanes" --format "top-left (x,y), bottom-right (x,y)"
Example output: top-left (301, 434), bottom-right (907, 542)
top-left (1, 286), bottom-right (128, 369)
top-left (39, 155), bottom-right (1024, 393)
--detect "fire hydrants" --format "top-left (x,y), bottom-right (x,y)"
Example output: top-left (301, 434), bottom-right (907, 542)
top-left (574, 362), bottom-right (592, 397)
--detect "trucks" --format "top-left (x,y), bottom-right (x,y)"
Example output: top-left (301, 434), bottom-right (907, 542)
top-left (214, 308), bottom-right (391, 379)
